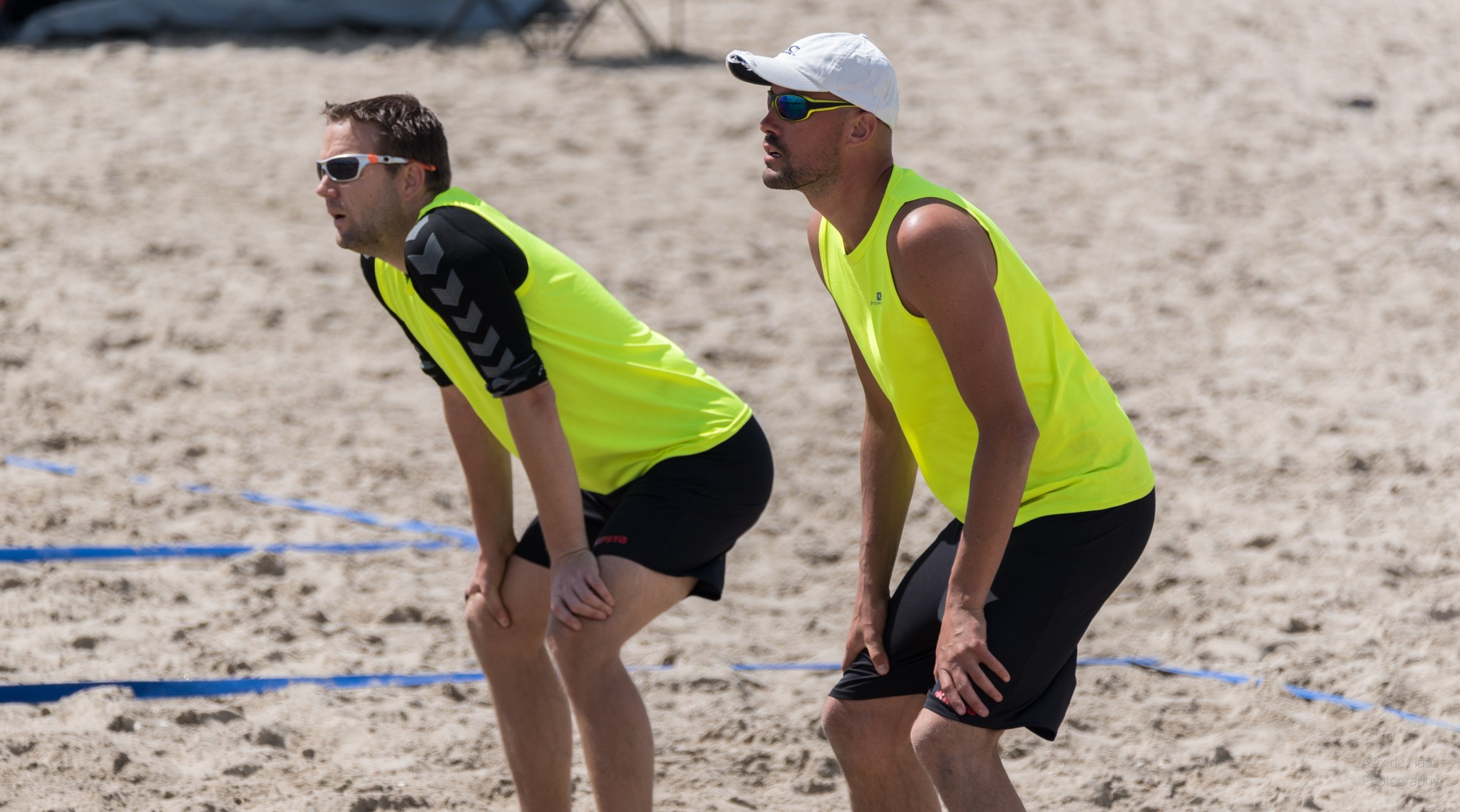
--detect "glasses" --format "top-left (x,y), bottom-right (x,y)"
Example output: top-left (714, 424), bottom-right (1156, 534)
top-left (766, 89), bottom-right (857, 122)
top-left (316, 152), bottom-right (437, 183)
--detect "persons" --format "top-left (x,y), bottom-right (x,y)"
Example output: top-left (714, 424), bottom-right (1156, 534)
top-left (727, 29), bottom-right (1156, 812)
top-left (311, 95), bottom-right (774, 812)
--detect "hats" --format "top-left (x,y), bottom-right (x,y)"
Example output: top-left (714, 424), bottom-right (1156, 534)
top-left (726, 31), bottom-right (901, 131)
